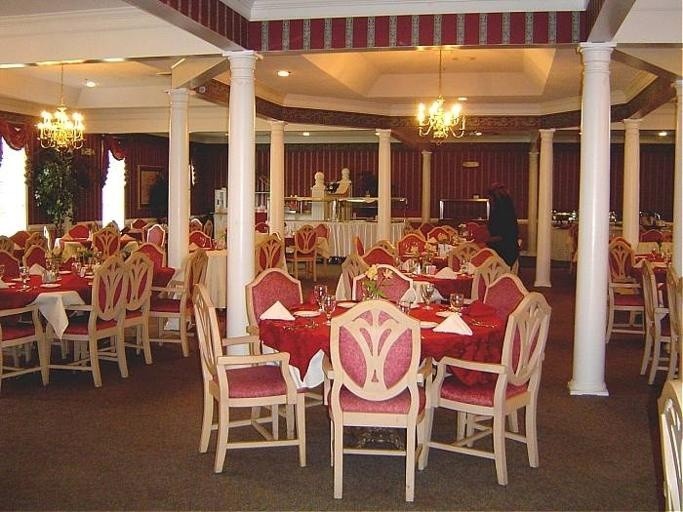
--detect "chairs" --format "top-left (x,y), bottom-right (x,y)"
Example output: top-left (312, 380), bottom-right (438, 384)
top-left (190, 284), bottom-right (308, 473)
top-left (564, 213), bottom-right (681, 389)
top-left (2, 218), bottom-right (226, 388)
top-left (323, 300), bottom-right (432, 503)
top-left (432, 292), bottom-right (553, 486)
top-left (340, 221), bottom-right (529, 442)
top-left (254, 221), bottom-right (331, 285)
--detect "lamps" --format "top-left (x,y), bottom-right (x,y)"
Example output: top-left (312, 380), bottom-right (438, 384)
top-left (35, 65), bottom-right (86, 155)
top-left (415, 50), bottom-right (466, 146)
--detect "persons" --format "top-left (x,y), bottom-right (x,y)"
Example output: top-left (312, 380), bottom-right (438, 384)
top-left (487, 183), bottom-right (518, 268)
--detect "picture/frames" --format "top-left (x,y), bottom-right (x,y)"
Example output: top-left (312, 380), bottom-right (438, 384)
top-left (137, 165), bottom-right (165, 212)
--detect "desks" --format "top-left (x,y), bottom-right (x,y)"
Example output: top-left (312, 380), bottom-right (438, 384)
top-left (406, 268), bottom-right (475, 300)
top-left (284, 218), bottom-right (406, 265)
top-left (54, 237), bottom-right (136, 268)
top-left (188, 249), bottom-right (233, 314)
top-left (0, 267), bottom-right (173, 378)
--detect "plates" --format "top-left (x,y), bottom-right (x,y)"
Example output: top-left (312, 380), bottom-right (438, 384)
top-left (420, 321), bottom-right (438, 329)
top-left (0, 255), bottom-right (99, 291)
top-left (292, 309), bottom-right (320, 318)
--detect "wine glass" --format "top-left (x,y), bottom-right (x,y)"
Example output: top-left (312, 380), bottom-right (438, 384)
top-left (651, 244), bottom-right (672, 265)
top-left (313, 282), bottom-right (464, 319)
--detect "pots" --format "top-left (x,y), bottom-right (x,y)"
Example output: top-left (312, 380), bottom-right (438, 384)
top-left (638, 208), bottom-right (662, 227)
